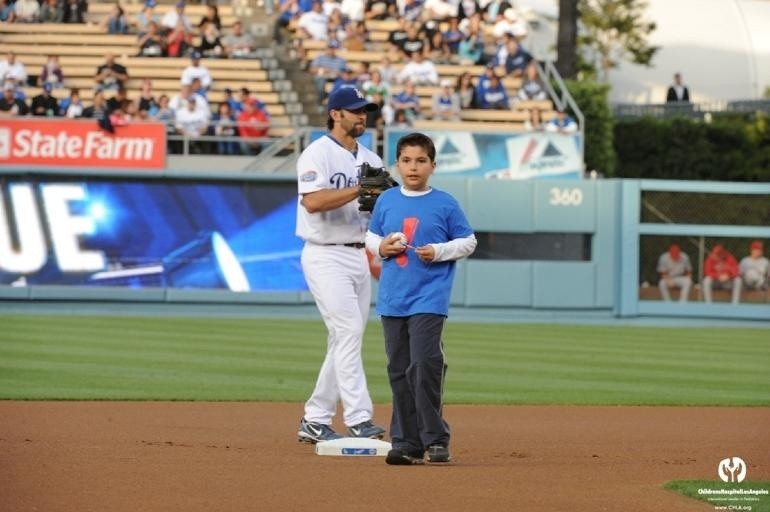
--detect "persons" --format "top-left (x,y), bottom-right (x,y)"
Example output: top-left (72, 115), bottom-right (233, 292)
top-left (701, 243), bottom-right (742, 302)
top-left (0, 0), bottom-right (578, 154)
top-left (657, 241), bottom-right (694, 302)
top-left (296, 87), bottom-right (398, 442)
top-left (737, 242), bottom-right (769, 290)
top-left (363, 132), bottom-right (478, 465)
top-left (667, 73), bottom-right (689, 103)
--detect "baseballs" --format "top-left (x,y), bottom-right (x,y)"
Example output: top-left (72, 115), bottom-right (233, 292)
top-left (391, 232), bottom-right (407, 248)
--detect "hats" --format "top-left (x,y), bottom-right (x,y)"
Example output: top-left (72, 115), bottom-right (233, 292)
top-left (329, 88), bottom-right (378, 111)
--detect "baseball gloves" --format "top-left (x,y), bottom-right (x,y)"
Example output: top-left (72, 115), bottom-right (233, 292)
top-left (357, 176), bottom-right (393, 211)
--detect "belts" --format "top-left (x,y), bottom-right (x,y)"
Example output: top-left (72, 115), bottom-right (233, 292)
top-left (327, 243), bottom-right (365, 248)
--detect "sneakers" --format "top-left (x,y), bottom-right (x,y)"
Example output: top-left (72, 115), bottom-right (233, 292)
top-left (386, 444), bottom-right (450, 465)
top-left (298, 417), bottom-right (340, 442)
top-left (346, 422), bottom-right (386, 440)
